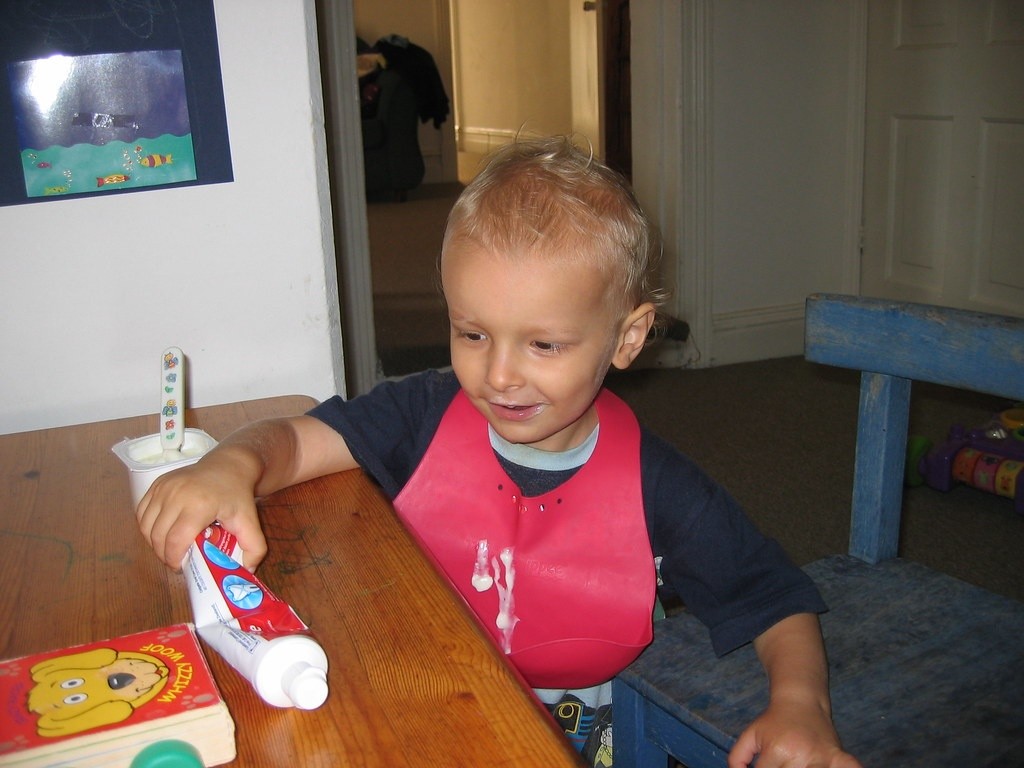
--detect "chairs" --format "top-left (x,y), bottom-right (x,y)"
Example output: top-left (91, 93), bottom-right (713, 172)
top-left (612, 291), bottom-right (1024, 768)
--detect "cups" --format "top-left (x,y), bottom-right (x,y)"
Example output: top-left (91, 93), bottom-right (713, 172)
top-left (112, 428), bottom-right (220, 514)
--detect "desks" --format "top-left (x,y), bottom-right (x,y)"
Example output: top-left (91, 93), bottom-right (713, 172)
top-left (1, 396), bottom-right (589, 768)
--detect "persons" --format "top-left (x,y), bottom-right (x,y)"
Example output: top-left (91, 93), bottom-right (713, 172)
top-left (133, 130), bottom-right (862, 768)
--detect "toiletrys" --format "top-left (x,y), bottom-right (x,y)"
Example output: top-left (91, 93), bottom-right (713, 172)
top-left (178, 521), bottom-right (334, 713)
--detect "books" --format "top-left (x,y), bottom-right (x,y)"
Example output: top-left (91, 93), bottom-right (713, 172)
top-left (0, 622), bottom-right (237, 768)
